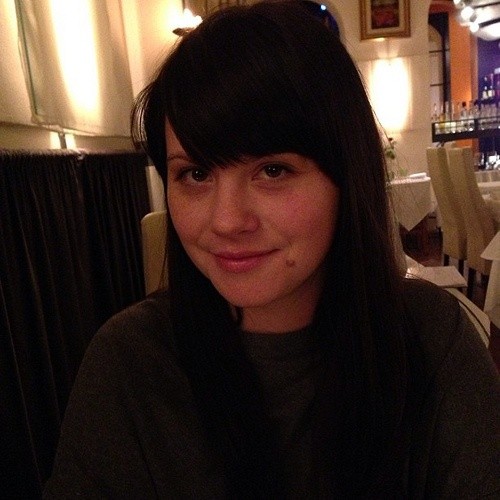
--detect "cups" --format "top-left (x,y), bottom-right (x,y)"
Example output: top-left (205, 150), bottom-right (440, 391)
top-left (487, 150), bottom-right (497, 170)
top-left (475, 152), bottom-right (485, 171)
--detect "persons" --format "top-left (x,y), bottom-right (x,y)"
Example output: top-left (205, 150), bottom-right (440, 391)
top-left (46, 1), bottom-right (500, 500)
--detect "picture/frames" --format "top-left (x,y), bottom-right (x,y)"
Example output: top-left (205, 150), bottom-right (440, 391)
top-left (357, 0), bottom-right (411, 40)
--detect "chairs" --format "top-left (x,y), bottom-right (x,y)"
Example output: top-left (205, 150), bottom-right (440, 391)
top-left (394, 145), bottom-right (500, 348)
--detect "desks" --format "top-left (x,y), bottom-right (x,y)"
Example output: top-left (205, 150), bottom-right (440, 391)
top-left (476, 179), bottom-right (500, 236)
top-left (388, 177), bottom-right (439, 256)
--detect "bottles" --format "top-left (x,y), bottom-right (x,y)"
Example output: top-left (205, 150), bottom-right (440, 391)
top-left (432, 100), bottom-right (500, 134)
top-left (487, 73), bottom-right (495, 100)
top-left (482, 76), bottom-right (489, 100)
